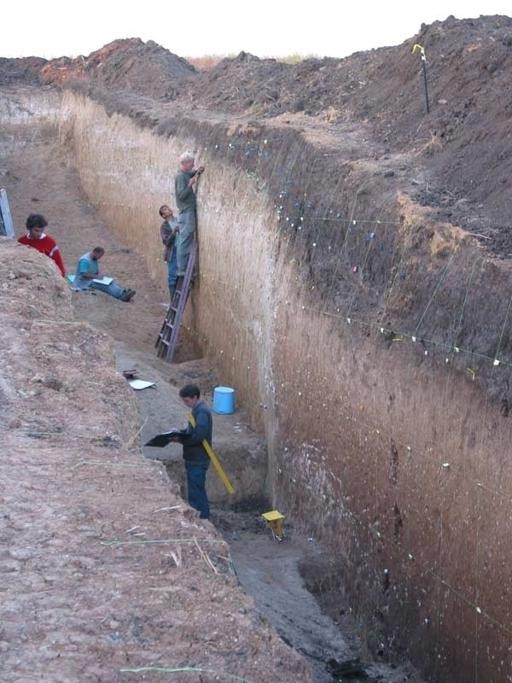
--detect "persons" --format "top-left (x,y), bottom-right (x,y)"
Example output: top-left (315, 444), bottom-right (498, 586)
top-left (72, 243), bottom-right (137, 303)
top-left (171, 382), bottom-right (214, 520)
top-left (157, 202), bottom-right (181, 299)
top-left (16, 211), bottom-right (66, 278)
top-left (172, 150), bottom-right (206, 292)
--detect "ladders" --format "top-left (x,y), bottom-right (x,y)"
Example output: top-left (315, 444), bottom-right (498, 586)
top-left (155, 242), bottom-right (197, 363)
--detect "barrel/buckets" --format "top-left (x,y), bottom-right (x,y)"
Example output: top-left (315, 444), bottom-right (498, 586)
top-left (213, 385), bottom-right (236, 415)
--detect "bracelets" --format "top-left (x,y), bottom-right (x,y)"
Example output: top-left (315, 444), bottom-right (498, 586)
top-left (92, 273), bottom-right (96, 278)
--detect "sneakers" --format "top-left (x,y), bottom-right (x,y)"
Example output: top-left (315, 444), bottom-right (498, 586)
top-left (119, 288), bottom-right (136, 302)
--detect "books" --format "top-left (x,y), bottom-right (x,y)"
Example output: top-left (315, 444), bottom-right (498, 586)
top-left (142, 430), bottom-right (191, 449)
top-left (93, 275), bottom-right (113, 285)
